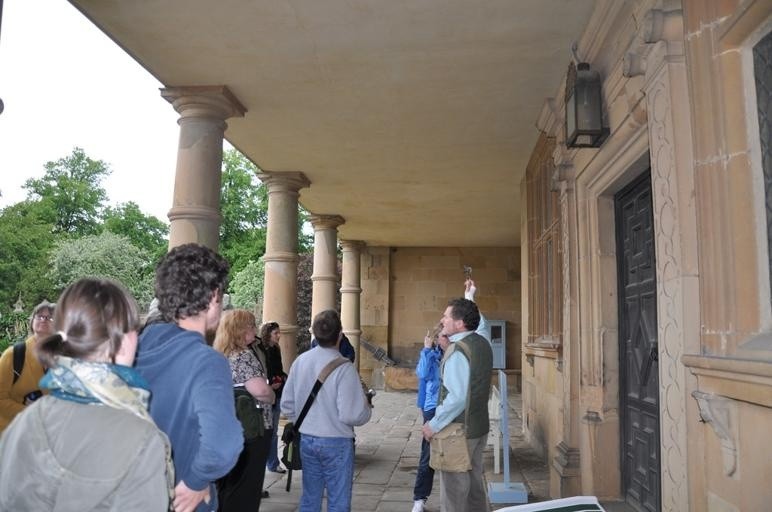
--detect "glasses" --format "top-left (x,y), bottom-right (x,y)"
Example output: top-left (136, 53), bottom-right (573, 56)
top-left (268, 322), bottom-right (277, 329)
top-left (33, 314), bottom-right (54, 323)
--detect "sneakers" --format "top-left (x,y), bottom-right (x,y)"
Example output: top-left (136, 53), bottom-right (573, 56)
top-left (412, 497), bottom-right (427, 512)
top-left (275, 465), bottom-right (286, 473)
top-left (261, 489), bottom-right (269, 497)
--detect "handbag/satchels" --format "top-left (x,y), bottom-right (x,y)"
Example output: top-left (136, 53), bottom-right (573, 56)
top-left (234, 386), bottom-right (264, 443)
top-left (428, 423), bottom-right (473, 473)
top-left (281, 423), bottom-right (302, 470)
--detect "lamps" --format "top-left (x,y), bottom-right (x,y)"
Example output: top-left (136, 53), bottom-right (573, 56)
top-left (563, 43), bottom-right (611, 153)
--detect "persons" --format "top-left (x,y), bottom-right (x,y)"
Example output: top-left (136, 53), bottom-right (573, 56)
top-left (310, 331), bottom-right (358, 366)
top-left (2, 298), bottom-right (67, 435)
top-left (272, 309), bottom-right (375, 512)
top-left (256, 320), bottom-right (289, 475)
top-left (1, 274), bottom-right (179, 510)
top-left (419, 279), bottom-right (497, 512)
top-left (125, 240), bottom-right (247, 511)
top-left (410, 322), bottom-right (456, 511)
top-left (205, 307), bottom-right (278, 511)
